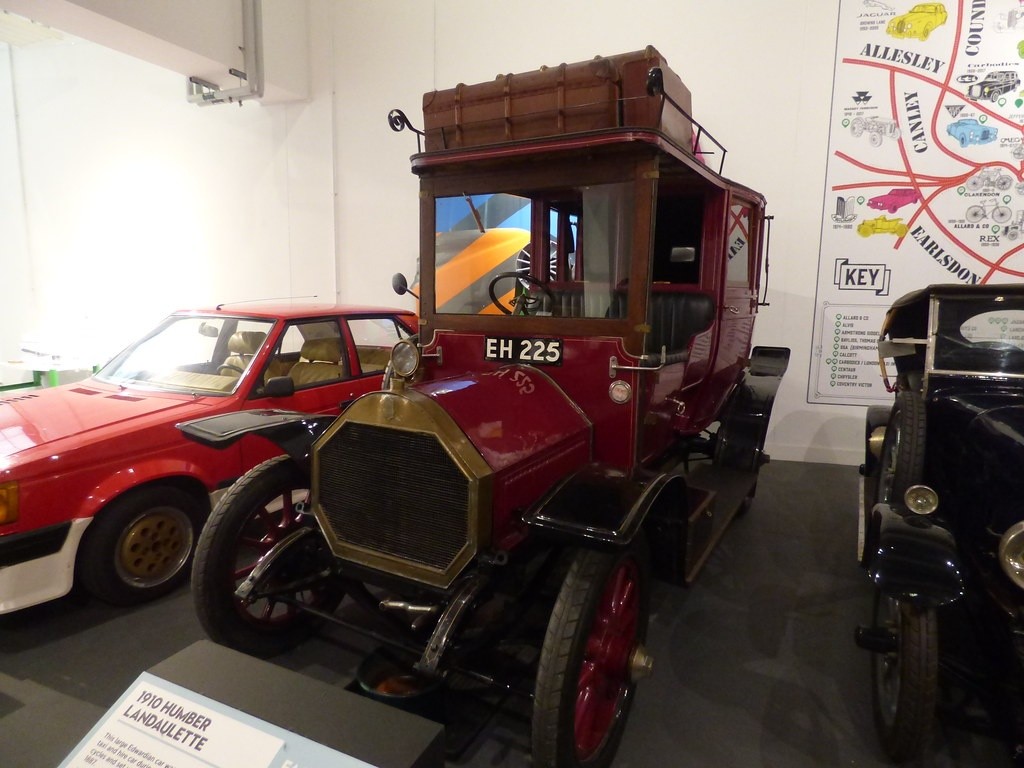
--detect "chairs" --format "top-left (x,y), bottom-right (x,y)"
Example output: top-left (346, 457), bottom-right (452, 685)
top-left (287, 337), bottom-right (344, 389)
top-left (220, 331), bottom-right (281, 385)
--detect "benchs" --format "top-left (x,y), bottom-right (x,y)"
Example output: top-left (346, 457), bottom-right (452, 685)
top-left (526, 291), bottom-right (717, 368)
top-left (301, 345), bottom-right (393, 374)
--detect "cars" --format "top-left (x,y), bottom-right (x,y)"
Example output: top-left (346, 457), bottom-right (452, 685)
top-left (0, 301), bottom-right (418, 619)
top-left (169, 46), bottom-right (793, 768)
top-left (855, 284), bottom-right (1023, 768)
